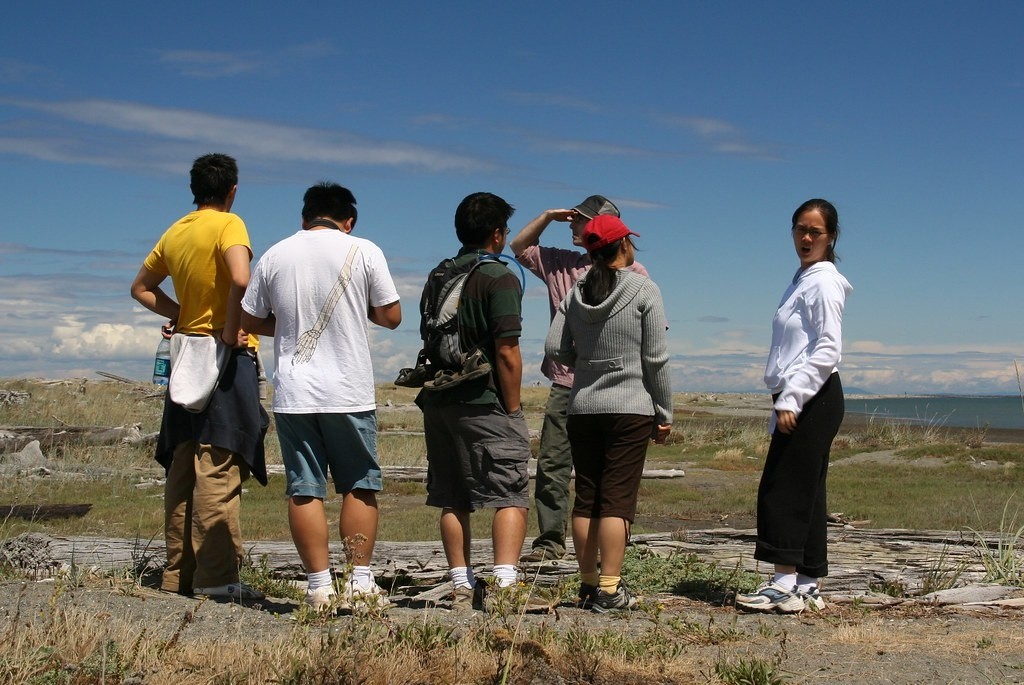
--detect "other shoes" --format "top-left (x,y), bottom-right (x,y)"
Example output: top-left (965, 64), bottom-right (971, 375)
top-left (519, 550), bottom-right (547, 562)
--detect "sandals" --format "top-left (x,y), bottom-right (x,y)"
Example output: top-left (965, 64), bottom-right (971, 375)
top-left (422, 348), bottom-right (493, 391)
top-left (393, 349), bottom-right (443, 387)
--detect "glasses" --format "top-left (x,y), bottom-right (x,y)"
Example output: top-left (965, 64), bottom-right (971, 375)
top-left (793, 225), bottom-right (831, 238)
top-left (505, 228), bottom-right (511, 235)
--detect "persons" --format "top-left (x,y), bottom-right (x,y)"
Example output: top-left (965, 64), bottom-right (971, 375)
top-left (130, 153), bottom-right (266, 602)
top-left (415, 191), bottom-right (562, 613)
top-left (543, 213), bottom-right (672, 616)
top-left (238, 182), bottom-right (400, 615)
top-left (509, 194), bottom-right (654, 562)
top-left (735, 198), bottom-right (846, 614)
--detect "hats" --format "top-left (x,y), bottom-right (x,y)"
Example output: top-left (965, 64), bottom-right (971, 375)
top-left (572, 196), bottom-right (621, 220)
top-left (582, 215), bottom-right (641, 253)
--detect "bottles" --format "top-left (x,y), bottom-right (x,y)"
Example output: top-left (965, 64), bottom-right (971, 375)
top-left (152, 330), bottom-right (172, 385)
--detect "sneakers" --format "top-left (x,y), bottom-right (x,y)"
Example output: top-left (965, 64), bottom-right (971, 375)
top-left (453, 579), bottom-right (490, 612)
top-left (578, 584), bottom-right (600, 609)
top-left (484, 582), bottom-right (560, 615)
top-left (735, 578), bottom-right (806, 612)
top-left (592, 586), bottom-right (639, 614)
top-left (338, 573), bottom-right (392, 614)
top-left (296, 586), bottom-right (337, 615)
top-left (799, 586), bottom-right (824, 610)
top-left (193, 582), bottom-right (266, 601)
